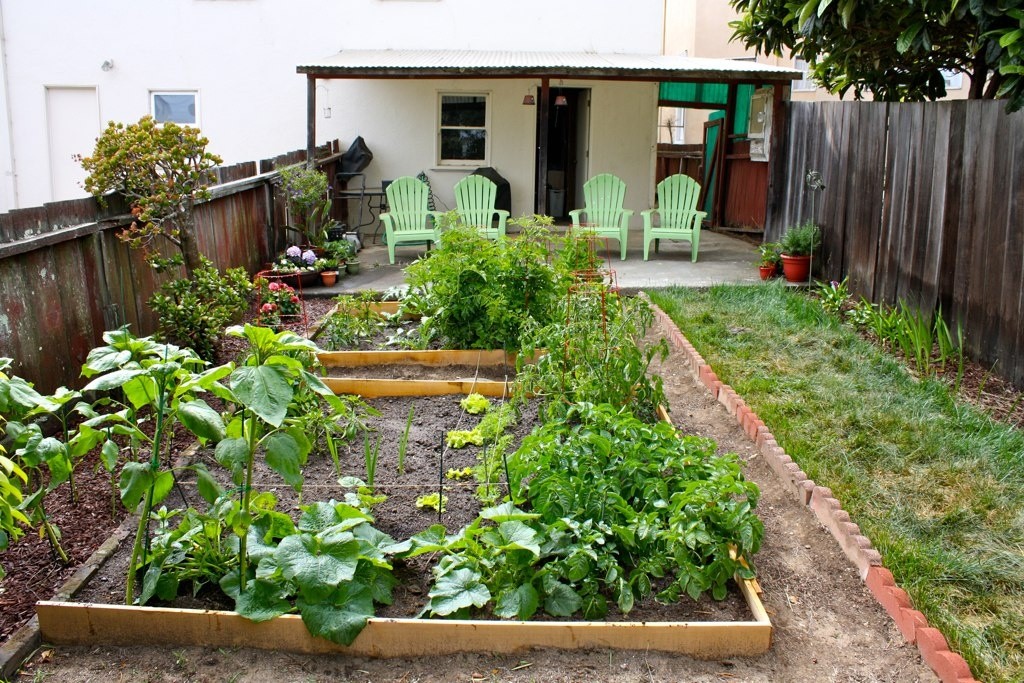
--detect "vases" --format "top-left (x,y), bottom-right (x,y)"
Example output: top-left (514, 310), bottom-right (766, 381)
top-left (256, 244), bottom-right (346, 290)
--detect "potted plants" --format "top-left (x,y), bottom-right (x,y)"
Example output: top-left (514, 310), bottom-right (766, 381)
top-left (336, 240), bottom-right (360, 279)
top-left (751, 218), bottom-right (822, 282)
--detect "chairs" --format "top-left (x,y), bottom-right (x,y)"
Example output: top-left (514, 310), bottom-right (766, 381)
top-left (379, 176), bottom-right (444, 265)
top-left (639, 174), bottom-right (708, 263)
top-left (450, 175), bottom-right (510, 250)
top-left (569, 173), bottom-right (634, 261)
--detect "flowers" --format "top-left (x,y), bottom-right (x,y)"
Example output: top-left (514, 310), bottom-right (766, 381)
top-left (264, 160), bottom-right (337, 275)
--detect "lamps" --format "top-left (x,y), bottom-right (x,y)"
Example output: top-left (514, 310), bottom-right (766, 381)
top-left (101, 58), bottom-right (113, 71)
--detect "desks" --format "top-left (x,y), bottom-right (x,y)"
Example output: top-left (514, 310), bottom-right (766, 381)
top-left (338, 188), bottom-right (390, 244)
